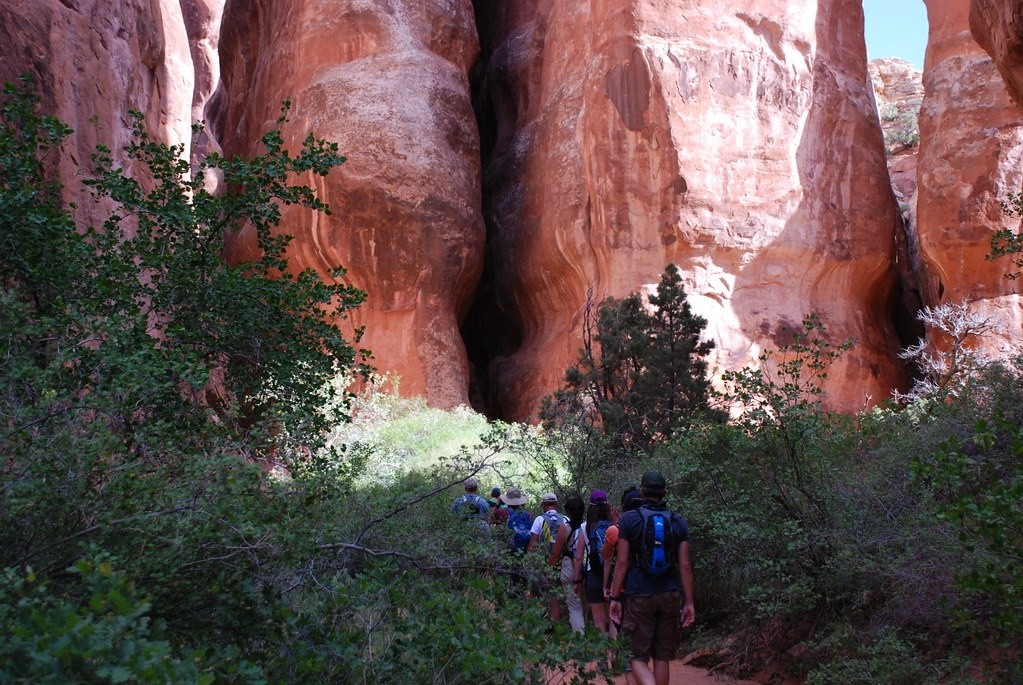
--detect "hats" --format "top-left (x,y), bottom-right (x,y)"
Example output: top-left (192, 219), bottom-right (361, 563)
top-left (540, 494), bottom-right (558, 506)
top-left (589, 489), bottom-right (607, 505)
top-left (499, 487), bottom-right (527, 505)
top-left (640, 469), bottom-right (665, 489)
top-left (465, 479), bottom-right (477, 489)
top-left (623, 490), bottom-right (645, 509)
top-left (492, 488), bottom-right (501, 496)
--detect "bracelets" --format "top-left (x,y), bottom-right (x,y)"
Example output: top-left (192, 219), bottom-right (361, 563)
top-left (574, 581), bottom-right (581, 584)
top-left (610, 596), bottom-right (620, 600)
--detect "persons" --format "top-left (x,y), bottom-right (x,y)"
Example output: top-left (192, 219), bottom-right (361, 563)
top-left (528, 493), bottom-right (571, 644)
top-left (574, 491), bottom-right (621, 675)
top-left (610, 472), bottom-right (695, 685)
top-left (602, 490), bottom-right (638, 685)
top-left (449, 479), bottom-right (535, 625)
top-left (545, 498), bottom-right (589, 659)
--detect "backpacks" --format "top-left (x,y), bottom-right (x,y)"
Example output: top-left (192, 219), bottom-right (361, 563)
top-left (633, 506), bottom-right (677, 577)
top-left (571, 529), bottom-right (588, 572)
top-left (462, 495), bottom-right (484, 522)
top-left (589, 520), bottom-right (614, 579)
top-left (504, 509), bottom-right (533, 549)
top-left (540, 513), bottom-right (564, 558)
